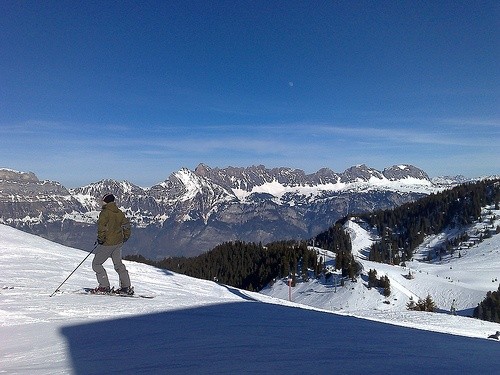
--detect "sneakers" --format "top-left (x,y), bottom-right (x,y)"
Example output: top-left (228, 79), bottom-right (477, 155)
top-left (86, 286), bottom-right (114, 295)
top-left (112, 287), bottom-right (135, 296)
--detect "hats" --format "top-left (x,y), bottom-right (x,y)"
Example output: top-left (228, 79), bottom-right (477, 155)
top-left (102, 193), bottom-right (115, 203)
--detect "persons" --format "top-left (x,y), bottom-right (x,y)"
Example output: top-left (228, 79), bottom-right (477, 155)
top-left (90, 193), bottom-right (134, 296)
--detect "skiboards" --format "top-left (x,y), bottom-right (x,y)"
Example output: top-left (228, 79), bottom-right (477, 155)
top-left (72, 291), bottom-right (156, 299)
